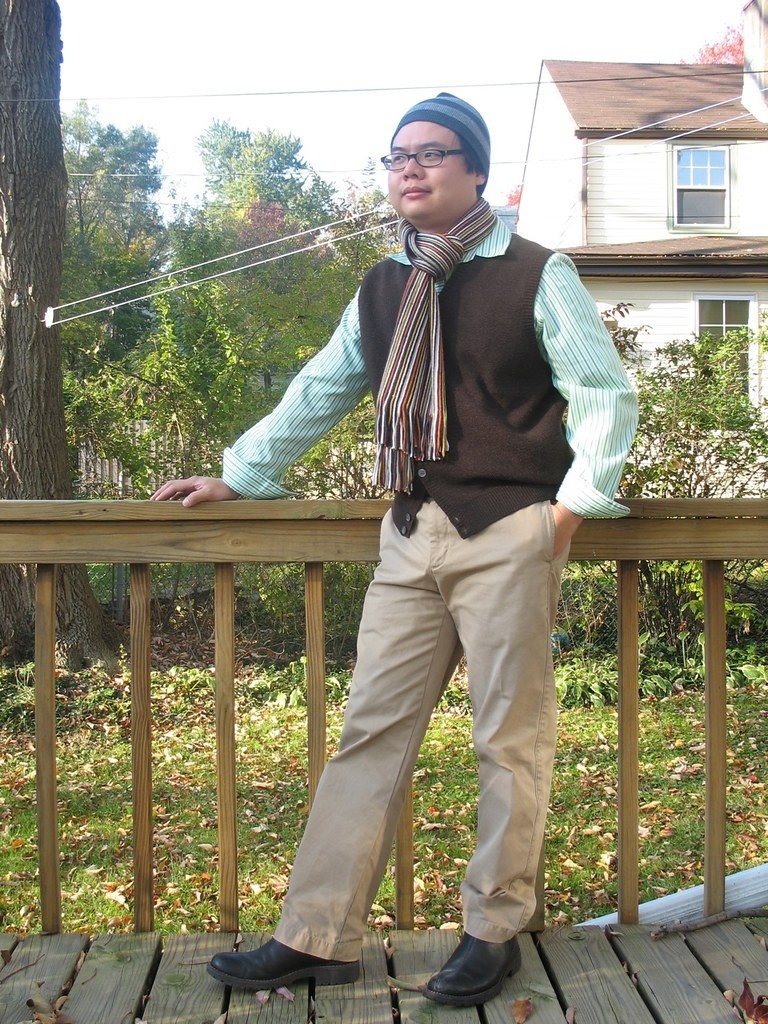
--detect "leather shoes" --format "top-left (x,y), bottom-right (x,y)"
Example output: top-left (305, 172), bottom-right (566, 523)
top-left (206, 938), bottom-right (360, 988)
top-left (423, 934), bottom-right (521, 1005)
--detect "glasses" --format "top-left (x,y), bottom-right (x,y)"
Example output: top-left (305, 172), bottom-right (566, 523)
top-left (379, 148), bottom-right (464, 171)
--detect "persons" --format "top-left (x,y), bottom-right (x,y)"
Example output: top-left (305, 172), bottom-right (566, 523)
top-left (150, 92), bottom-right (638, 1007)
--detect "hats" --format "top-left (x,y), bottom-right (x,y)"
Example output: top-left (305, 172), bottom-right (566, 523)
top-left (390, 93), bottom-right (491, 196)
